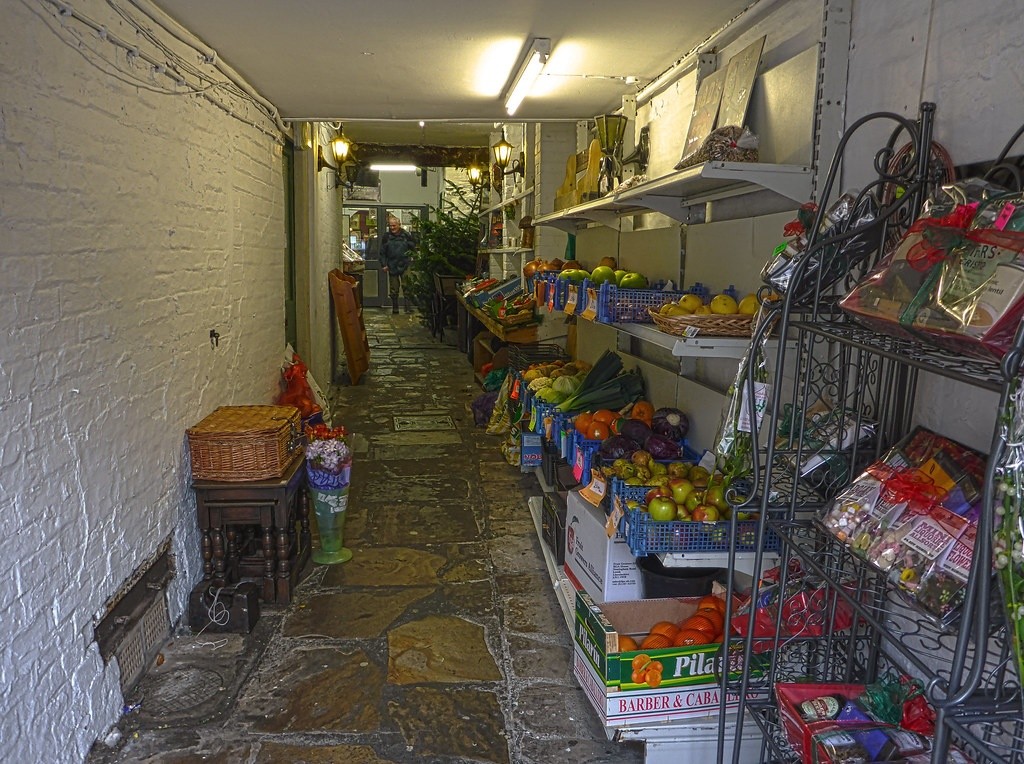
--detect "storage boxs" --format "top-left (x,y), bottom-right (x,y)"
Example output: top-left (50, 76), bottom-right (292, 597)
top-left (186, 405), bottom-right (304, 483)
top-left (803, 718), bottom-right (978, 762)
top-left (647, 304), bottom-right (784, 340)
top-left (562, 490), bottom-right (647, 607)
top-left (520, 381), bottom-right (786, 554)
top-left (525, 269), bottom-right (736, 324)
top-left (775, 681), bottom-right (902, 758)
top-left (572, 588), bottom-right (775, 728)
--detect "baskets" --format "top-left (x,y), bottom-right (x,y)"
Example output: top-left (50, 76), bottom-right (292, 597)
top-left (529, 265), bottom-right (780, 337)
top-left (185, 405), bottom-right (304, 482)
top-left (505, 341), bottom-right (782, 557)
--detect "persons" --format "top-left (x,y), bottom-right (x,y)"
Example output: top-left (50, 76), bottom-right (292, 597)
top-left (378, 216), bottom-right (416, 314)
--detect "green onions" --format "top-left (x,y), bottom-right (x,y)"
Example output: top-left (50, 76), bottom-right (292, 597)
top-left (540, 344), bottom-right (646, 413)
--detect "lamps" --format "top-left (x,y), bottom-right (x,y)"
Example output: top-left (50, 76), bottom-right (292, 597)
top-left (504, 37), bottom-right (551, 116)
top-left (331, 122), bottom-right (360, 194)
top-left (467, 154), bottom-right (490, 195)
top-left (370, 165), bottom-right (419, 171)
top-left (492, 125), bottom-right (524, 180)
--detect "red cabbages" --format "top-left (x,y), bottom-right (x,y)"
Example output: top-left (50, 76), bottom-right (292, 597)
top-left (605, 406), bottom-right (690, 459)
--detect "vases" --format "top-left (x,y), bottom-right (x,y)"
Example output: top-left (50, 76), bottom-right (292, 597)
top-left (312, 486), bottom-right (353, 564)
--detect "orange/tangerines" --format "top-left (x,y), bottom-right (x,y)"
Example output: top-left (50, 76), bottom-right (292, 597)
top-left (618, 596), bottom-right (731, 651)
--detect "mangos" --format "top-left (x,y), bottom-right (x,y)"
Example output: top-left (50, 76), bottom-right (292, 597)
top-left (661, 294), bottom-right (762, 316)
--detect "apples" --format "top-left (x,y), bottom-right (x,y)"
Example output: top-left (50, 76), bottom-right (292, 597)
top-left (559, 264), bottom-right (645, 289)
top-left (627, 479), bottom-right (767, 546)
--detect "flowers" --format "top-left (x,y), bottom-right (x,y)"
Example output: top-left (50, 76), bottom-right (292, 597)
top-left (305, 422), bottom-right (353, 489)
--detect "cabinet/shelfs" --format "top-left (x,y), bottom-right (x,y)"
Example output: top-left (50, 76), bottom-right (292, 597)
top-left (189, 454), bottom-right (312, 608)
top-left (330, 268), bottom-right (371, 386)
top-left (456, 122), bottom-right (1024, 764)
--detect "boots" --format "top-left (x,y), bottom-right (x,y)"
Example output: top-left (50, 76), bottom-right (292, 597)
top-left (404, 298), bottom-right (415, 312)
top-left (392, 299), bottom-right (399, 314)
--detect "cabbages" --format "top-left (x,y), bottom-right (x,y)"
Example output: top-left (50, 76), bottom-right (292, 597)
top-left (535, 371), bottom-right (588, 406)
